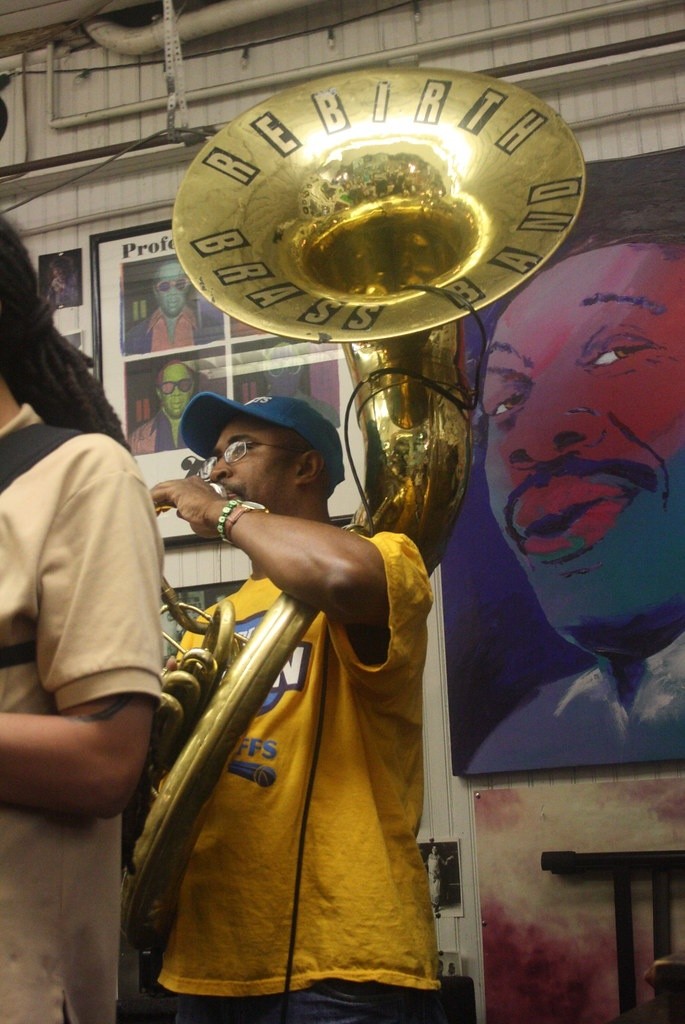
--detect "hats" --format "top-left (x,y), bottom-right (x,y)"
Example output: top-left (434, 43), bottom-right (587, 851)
top-left (180, 391), bottom-right (345, 498)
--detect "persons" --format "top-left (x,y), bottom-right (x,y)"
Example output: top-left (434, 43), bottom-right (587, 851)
top-left (0, 217), bottom-right (164, 1024)
top-left (46, 255), bottom-right (82, 307)
top-left (126, 262), bottom-right (222, 356)
top-left (150, 391), bottom-right (441, 1024)
top-left (423, 846), bottom-right (454, 912)
top-left (130, 341), bottom-right (341, 460)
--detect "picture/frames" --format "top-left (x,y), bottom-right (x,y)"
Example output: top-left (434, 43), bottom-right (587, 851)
top-left (89, 219), bottom-right (354, 549)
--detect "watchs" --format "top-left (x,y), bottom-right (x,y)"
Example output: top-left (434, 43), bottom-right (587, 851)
top-left (225, 501), bottom-right (270, 542)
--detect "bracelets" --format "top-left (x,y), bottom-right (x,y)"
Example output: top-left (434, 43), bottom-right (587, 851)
top-left (217, 500), bottom-right (242, 544)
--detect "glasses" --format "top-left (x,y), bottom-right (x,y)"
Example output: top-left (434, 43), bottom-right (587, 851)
top-left (197, 439), bottom-right (308, 482)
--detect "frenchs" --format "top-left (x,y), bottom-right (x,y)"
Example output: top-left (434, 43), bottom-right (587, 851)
top-left (115, 55), bottom-right (590, 958)
